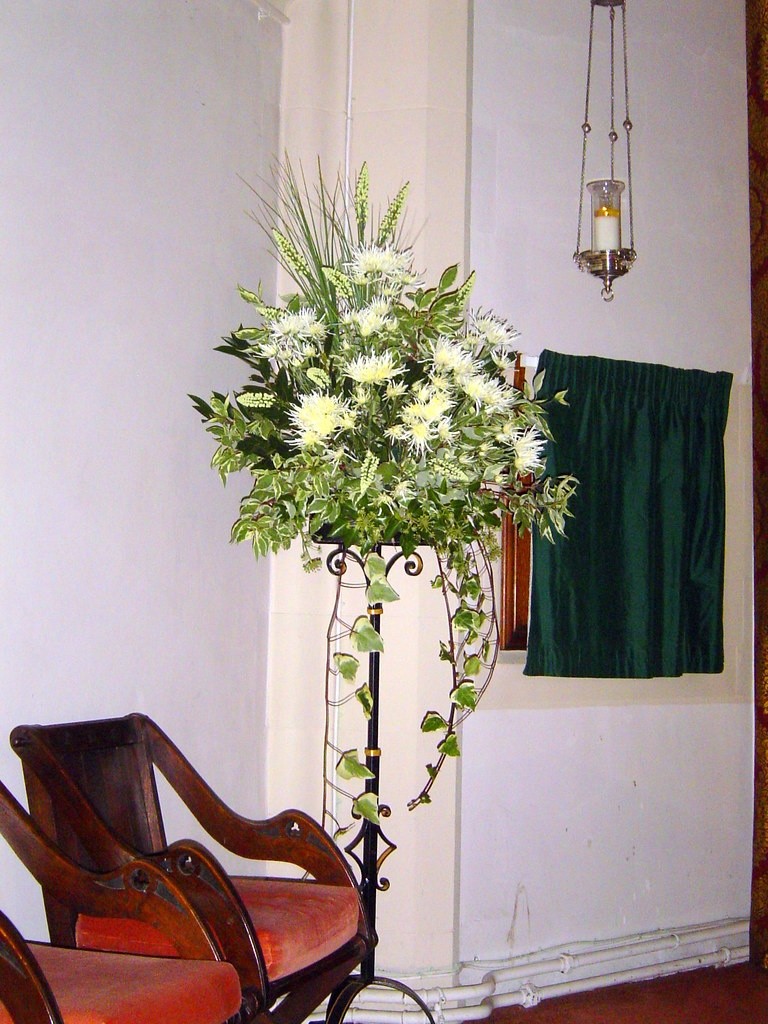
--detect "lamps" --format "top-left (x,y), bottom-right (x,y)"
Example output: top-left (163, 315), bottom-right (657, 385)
top-left (573, 0), bottom-right (638, 302)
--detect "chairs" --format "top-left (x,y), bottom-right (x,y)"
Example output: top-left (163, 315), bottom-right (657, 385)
top-left (10, 712), bottom-right (378, 1024)
top-left (0, 781), bottom-right (258, 1024)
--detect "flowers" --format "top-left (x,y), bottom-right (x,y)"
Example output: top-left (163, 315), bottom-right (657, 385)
top-left (184, 146), bottom-right (588, 882)
